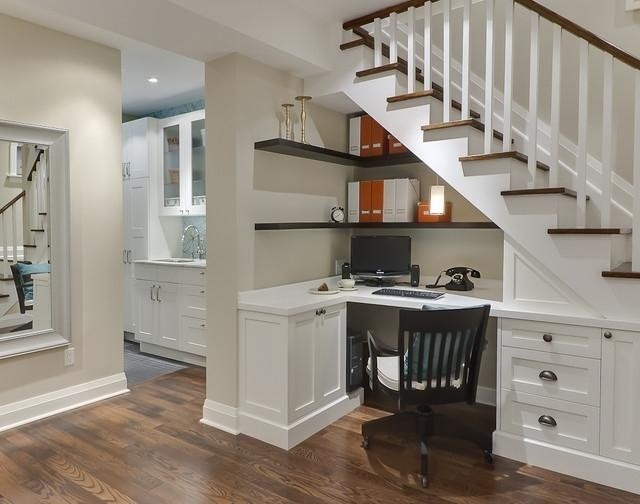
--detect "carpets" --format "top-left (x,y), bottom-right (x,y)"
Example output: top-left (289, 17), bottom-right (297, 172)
top-left (124, 341), bottom-right (186, 386)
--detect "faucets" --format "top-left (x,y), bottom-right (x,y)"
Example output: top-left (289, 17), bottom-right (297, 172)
top-left (181, 224), bottom-right (200, 246)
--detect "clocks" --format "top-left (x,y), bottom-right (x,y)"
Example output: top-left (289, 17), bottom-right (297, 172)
top-left (331, 207), bottom-right (344, 222)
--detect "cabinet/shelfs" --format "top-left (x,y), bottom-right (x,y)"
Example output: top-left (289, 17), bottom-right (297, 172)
top-left (121, 117), bottom-right (182, 343)
top-left (494, 317), bottom-right (640, 496)
top-left (255, 139), bottom-right (501, 229)
top-left (238, 303), bottom-right (347, 451)
top-left (135, 263), bottom-right (206, 368)
top-left (160, 107), bottom-right (205, 216)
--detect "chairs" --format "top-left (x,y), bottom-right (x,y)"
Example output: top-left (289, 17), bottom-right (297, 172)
top-left (360, 303), bottom-right (491, 487)
top-left (11, 263), bottom-right (33, 314)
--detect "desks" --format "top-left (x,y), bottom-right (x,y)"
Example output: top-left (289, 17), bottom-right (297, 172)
top-left (239, 275), bottom-right (503, 317)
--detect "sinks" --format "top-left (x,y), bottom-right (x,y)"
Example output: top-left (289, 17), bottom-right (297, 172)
top-left (153, 258), bottom-right (196, 262)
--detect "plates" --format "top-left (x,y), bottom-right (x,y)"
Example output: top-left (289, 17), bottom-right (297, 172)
top-left (309, 287), bottom-right (340, 295)
top-left (338, 287), bottom-right (359, 291)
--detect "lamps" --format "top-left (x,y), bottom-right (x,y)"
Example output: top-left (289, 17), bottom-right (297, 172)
top-left (430, 175), bottom-right (445, 214)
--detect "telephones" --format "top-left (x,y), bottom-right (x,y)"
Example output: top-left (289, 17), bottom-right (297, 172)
top-left (445, 267), bottom-right (480, 291)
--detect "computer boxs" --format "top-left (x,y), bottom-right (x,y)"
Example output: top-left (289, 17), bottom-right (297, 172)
top-left (347, 331), bottom-right (364, 392)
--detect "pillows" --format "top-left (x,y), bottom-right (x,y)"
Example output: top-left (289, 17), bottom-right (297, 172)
top-left (403, 305), bottom-right (485, 381)
top-left (18, 263), bottom-right (50, 300)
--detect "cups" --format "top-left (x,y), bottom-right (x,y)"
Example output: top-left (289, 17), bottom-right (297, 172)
top-left (336, 278), bottom-right (356, 288)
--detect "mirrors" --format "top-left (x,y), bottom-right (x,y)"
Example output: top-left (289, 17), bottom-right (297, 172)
top-left (1, 121), bottom-right (72, 359)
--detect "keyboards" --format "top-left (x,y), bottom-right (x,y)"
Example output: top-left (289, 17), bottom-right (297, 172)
top-left (372, 289), bottom-right (445, 298)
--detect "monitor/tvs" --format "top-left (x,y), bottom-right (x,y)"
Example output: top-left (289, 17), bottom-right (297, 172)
top-left (351, 235), bottom-right (409, 287)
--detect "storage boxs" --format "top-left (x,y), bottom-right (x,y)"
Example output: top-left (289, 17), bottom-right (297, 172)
top-left (418, 201), bottom-right (452, 222)
